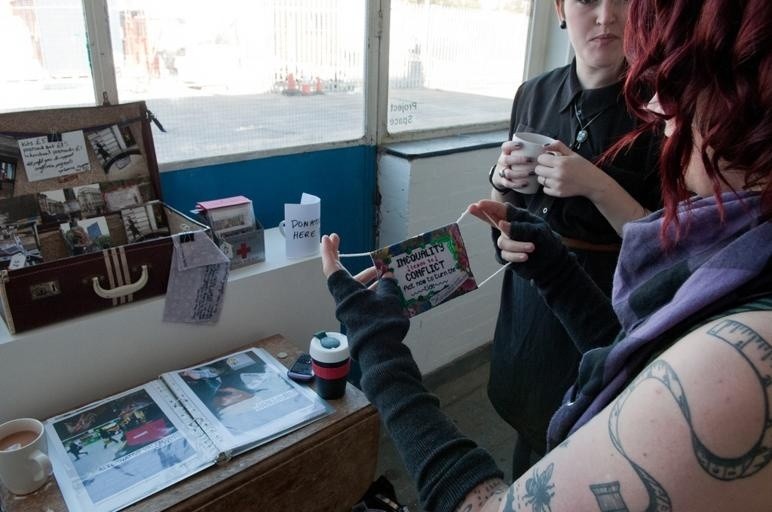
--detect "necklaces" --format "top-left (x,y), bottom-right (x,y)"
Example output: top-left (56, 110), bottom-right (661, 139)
top-left (573, 102), bottom-right (606, 146)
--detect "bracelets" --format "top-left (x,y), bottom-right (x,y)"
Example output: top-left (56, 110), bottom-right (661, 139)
top-left (489, 163), bottom-right (510, 195)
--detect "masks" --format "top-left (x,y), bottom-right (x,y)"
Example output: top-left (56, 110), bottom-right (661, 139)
top-left (335, 200), bottom-right (516, 321)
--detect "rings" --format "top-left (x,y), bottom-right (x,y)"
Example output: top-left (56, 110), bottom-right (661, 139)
top-left (500, 167), bottom-right (508, 177)
top-left (543, 176), bottom-right (548, 186)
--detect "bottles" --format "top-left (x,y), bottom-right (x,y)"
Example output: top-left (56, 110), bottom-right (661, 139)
top-left (310, 330), bottom-right (351, 400)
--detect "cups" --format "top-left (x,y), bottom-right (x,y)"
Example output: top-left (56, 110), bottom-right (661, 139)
top-left (0, 417), bottom-right (53, 496)
top-left (510, 131), bottom-right (563, 195)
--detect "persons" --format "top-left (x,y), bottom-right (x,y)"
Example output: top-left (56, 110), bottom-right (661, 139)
top-left (320, 0), bottom-right (772, 512)
top-left (68, 442), bottom-right (90, 460)
top-left (99, 428), bottom-right (119, 446)
top-left (94, 139), bottom-right (111, 160)
top-left (126, 215), bottom-right (142, 238)
top-left (482, 2), bottom-right (698, 482)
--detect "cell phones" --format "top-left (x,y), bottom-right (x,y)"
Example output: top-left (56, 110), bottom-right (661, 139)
top-left (287, 352), bottom-right (316, 383)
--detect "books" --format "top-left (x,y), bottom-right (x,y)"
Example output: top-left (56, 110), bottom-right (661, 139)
top-left (40, 345), bottom-right (339, 511)
